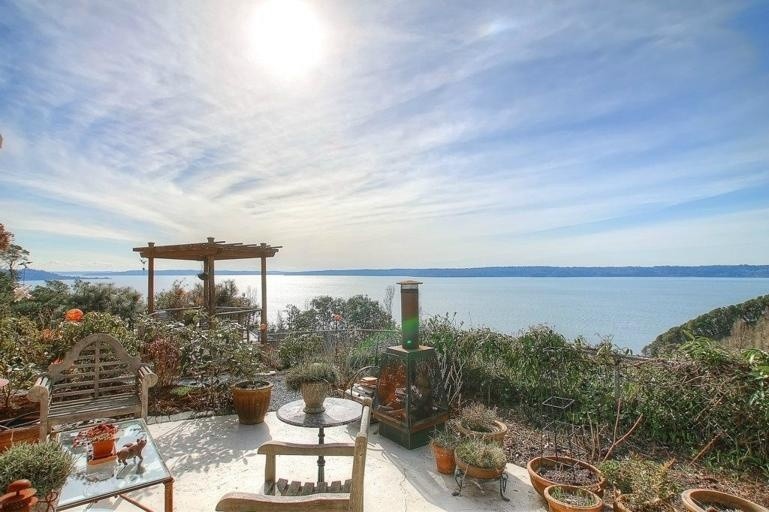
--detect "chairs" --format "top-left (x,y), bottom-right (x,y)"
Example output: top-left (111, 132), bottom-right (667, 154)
top-left (28, 331), bottom-right (160, 434)
top-left (212, 398), bottom-right (373, 512)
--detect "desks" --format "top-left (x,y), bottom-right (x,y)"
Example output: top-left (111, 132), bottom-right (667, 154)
top-left (276, 395), bottom-right (363, 483)
top-left (42, 420), bottom-right (175, 511)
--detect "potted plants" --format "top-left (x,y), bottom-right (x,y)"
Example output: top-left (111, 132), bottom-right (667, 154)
top-left (452, 401), bottom-right (509, 449)
top-left (613, 448), bottom-right (683, 512)
top-left (224, 343), bottom-right (274, 424)
top-left (284, 359), bottom-right (338, 416)
top-left (453, 440), bottom-right (514, 502)
top-left (431, 423), bottom-right (463, 476)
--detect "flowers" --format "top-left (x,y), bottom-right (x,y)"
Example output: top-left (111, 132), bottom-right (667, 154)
top-left (67, 423), bottom-right (120, 450)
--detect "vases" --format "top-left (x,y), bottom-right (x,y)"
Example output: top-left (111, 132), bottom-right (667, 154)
top-left (677, 476), bottom-right (768, 512)
top-left (542, 482), bottom-right (603, 512)
top-left (544, 419), bottom-right (581, 438)
top-left (527, 451), bottom-right (609, 496)
top-left (91, 440), bottom-right (116, 463)
top-left (542, 396), bottom-right (576, 415)
